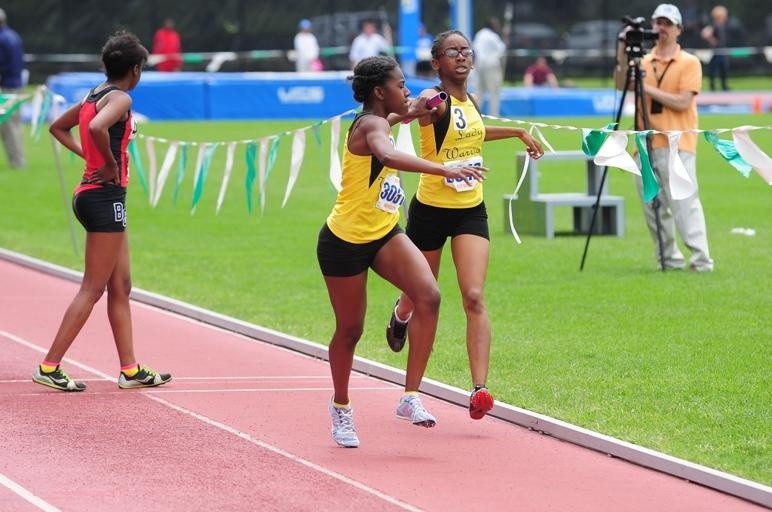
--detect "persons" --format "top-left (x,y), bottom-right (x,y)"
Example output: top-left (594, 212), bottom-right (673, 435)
top-left (614, 3), bottom-right (714, 275)
top-left (288, 18), bottom-right (323, 71)
top-left (316, 54), bottom-right (492, 451)
top-left (385, 26), bottom-right (546, 423)
top-left (523, 53), bottom-right (560, 89)
top-left (469, 17), bottom-right (506, 117)
top-left (414, 24), bottom-right (436, 80)
top-left (701, 5), bottom-right (730, 90)
top-left (152, 17), bottom-right (182, 71)
top-left (0, 9), bottom-right (27, 169)
top-left (30, 32), bottom-right (174, 391)
top-left (349, 20), bottom-right (390, 67)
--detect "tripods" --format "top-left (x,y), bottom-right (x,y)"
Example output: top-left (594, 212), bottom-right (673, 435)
top-left (580, 52), bottom-right (665, 273)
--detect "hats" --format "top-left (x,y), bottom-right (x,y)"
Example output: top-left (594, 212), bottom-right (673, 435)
top-left (652, 3), bottom-right (683, 25)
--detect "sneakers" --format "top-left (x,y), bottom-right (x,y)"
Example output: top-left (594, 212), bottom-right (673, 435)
top-left (387, 298), bottom-right (412, 352)
top-left (395, 395), bottom-right (436, 428)
top-left (118, 364), bottom-right (172, 389)
top-left (470, 387), bottom-right (494, 420)
top-left (328, 394), bottom-right (360, 447)
top-left (31, 365), bottom-right (86, 391)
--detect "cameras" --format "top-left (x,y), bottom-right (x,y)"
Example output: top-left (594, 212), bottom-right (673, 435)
top-left (622, 15), bottom-right (659, 51)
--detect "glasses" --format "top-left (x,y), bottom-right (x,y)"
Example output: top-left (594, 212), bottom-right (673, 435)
top-left (437, 47), bottom-right (475, 58)
